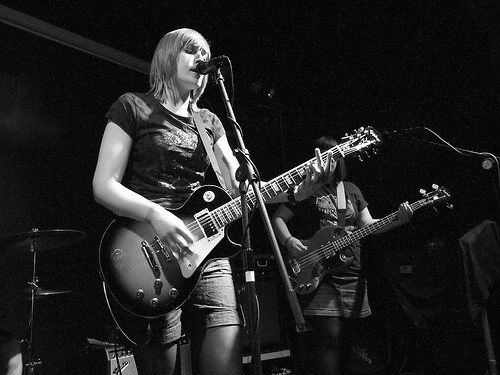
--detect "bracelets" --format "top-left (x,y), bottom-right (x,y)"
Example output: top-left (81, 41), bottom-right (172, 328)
top-left (287, 186), bottom-right (301, 205)
top-left (285, 236), bottom-right (293, 247)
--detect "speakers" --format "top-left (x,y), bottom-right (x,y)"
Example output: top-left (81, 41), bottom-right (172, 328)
top-left (86, 345), bottom-right (139, 375)
top-left (231, 255), bottom-right (283, 346)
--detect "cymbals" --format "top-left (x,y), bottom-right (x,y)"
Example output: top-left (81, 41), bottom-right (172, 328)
top-left (37, 289), bottom-right (72, 296)
top-left (0, 229), bottom-right (88, 254)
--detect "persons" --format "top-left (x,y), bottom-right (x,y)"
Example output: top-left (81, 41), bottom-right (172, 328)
top-left (93, 28), bottom-right (336, 375)
top-left (273, 136), bottom-right (414, 375)
top-left (0, 314), bottom-right (23, 375)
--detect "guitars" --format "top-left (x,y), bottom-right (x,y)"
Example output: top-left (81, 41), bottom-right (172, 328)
top-left (284, 182), bottom-right (454, 296)
top-left (98, 126), bottom-right (384, 319)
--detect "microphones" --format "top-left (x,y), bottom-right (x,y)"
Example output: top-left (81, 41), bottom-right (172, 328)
top-left (198, 55), bottom-right (225, 75)
top-left (482, 159), bottom-right (493, 170)
